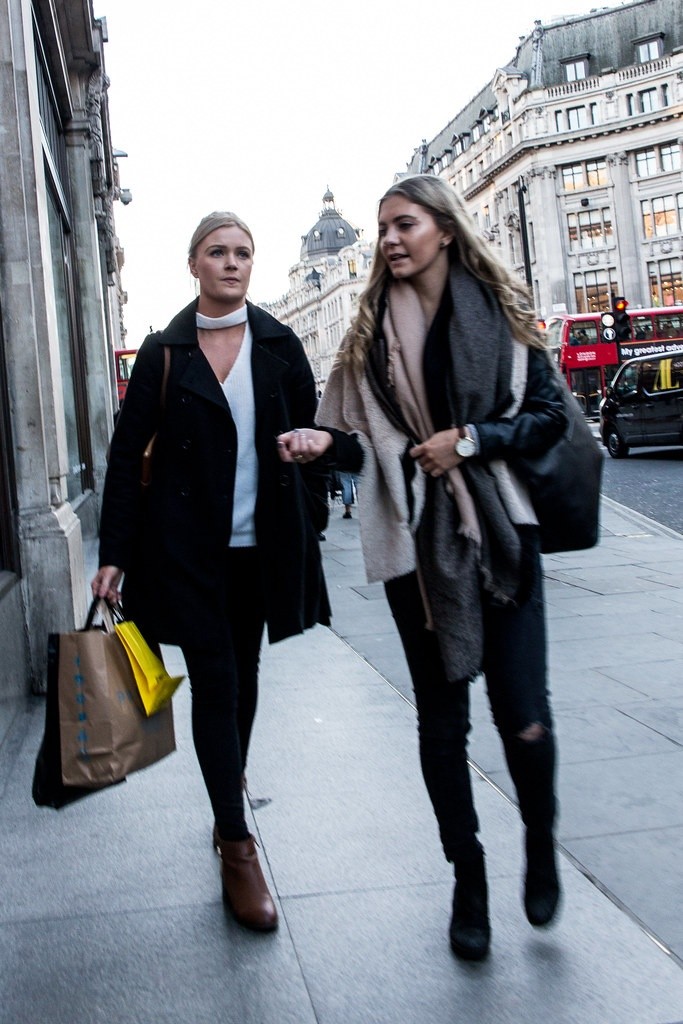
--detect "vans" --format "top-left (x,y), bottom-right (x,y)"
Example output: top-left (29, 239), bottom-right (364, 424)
top-left (598, 349), bottom-right (683, 459)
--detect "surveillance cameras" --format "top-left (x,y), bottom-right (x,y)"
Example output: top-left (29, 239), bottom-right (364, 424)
top-left (120, 193), bottom-right (132, 205)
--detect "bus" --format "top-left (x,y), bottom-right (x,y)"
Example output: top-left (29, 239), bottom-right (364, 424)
top-left (542, 305), bottom-right (683, 422)
top-left (114, 348), bottom-right (139, 408)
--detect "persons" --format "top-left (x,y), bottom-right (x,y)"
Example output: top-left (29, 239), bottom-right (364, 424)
top-left (277, 176), bottom-right (565, 962)
top-left (318, 470), bottom-right (360, 541)
top-left (569, 328), bottom-right (588, 346)
top-left (634, 321), bottom-right (677, 342)
top-left (90, 212), bottom-right (332, 934)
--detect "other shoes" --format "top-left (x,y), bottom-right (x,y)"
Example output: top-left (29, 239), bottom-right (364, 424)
top-left (342, 513), bottom-right (352, 520)
top-left (524, 830), bottom-right (558, 926)
top-left (450, 864), bottom-right (490, 961)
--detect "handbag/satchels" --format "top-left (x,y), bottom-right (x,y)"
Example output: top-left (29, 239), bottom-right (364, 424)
top-left (106, 399), bottom-right (157, 490)
top-left (506, 361), bottom-right (604, 554)
top-left (32, 593), bottom-right (185, 811)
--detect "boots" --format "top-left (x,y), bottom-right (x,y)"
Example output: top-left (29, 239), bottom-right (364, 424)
top-left (214, 822), bottom-right (278, 932)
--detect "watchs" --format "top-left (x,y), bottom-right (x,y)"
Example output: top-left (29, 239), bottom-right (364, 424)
top-left (454, 427), bottom-right (476, 458)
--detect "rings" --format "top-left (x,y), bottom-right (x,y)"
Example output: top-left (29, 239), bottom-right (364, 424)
top-left (293, 453), bottom-right (303, 462)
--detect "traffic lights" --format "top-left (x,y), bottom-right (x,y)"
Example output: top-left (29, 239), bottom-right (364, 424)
top-left (599, 297), bottom-right (633, 344)
